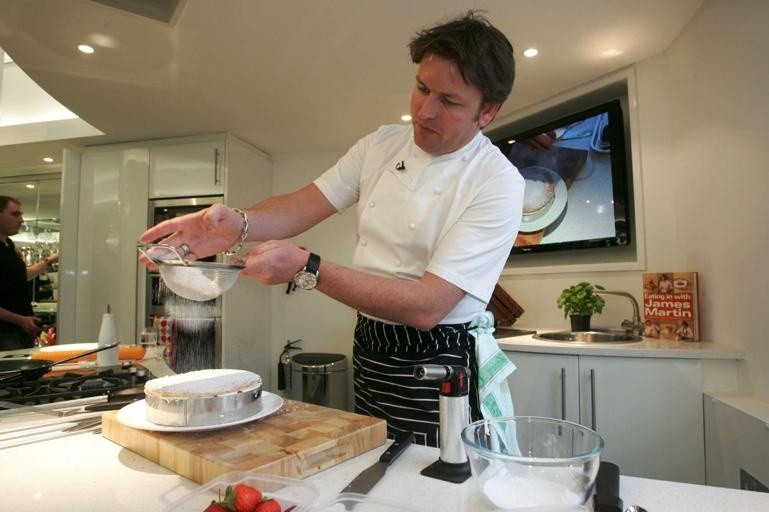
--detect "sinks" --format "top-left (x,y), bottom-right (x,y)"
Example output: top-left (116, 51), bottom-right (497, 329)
top-left (532, 331), bottom-right (644, 345)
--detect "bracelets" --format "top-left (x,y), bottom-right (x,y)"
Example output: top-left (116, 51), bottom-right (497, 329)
top-left (220, 208), bottom-right (248, 256)
top-left (45, 258), bottom-right (52, 269)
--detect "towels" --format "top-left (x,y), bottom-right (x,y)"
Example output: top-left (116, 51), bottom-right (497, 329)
top-left (468, 311), bottom-right (523, 457)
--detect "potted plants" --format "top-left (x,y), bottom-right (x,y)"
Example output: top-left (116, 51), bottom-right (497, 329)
top-left (556, 283), bottom-right (606, 332)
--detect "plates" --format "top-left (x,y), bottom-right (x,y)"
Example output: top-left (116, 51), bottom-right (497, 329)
top-left (320, 491), bottom-right (419, 511)
top-left (511, 165), bottom-right (569, 233)
top-left (163, 469), bottom-right (319, 512)
top-left (31, 341), bottom-right (132, 370)
top-left (117, 390), bottom-right (283, 431)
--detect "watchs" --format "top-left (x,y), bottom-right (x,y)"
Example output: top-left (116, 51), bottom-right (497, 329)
top-left (294, 253), bottom-right (321, 291)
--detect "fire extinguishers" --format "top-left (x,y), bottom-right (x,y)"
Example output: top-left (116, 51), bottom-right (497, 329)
top-left (277, 338), bottom-right (303, 396)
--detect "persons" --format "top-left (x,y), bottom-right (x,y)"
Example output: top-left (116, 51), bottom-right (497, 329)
top-left (644, 320), bottom-right (660, 338)
top-left (0, 195), bottom-right (59, 352)
top-left (644, 274), bottom-right (673, 293)
top-left (676, 321), bottom-right (694, 339)
top-left (135, 9), bottom-right (526, 459)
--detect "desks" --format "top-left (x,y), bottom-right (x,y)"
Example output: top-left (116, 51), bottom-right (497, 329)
top-left (0, 345), bottom-right (721, 507)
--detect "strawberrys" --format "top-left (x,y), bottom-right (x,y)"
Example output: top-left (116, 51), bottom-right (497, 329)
top-left (214, 484), bottom-right (262, 512)
top-left (255, 498), bottom-right (281, 512)
top-left (203, 500), bottom-right (230, 512)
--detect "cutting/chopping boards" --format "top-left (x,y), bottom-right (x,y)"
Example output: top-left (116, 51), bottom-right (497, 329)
top-left (101, 393), bottom-right (387, 497)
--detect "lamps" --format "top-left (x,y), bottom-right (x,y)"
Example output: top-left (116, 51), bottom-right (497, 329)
top-left (76, 43), bottom-right (95, 54)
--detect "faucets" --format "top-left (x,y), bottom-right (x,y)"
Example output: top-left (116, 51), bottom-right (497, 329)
top-left (592, 290), bottom-right (645, 336)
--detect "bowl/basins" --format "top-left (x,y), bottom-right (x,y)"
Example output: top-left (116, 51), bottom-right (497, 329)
top-left (461, 415), bottom-right (604, 511)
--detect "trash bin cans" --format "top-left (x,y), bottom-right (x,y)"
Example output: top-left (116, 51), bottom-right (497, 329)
top-left (289, 353), bottom-right (349, 412)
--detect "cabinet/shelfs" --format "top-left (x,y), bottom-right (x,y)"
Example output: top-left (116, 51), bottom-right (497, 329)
top-left (485, 338), bottom-right (721, 487)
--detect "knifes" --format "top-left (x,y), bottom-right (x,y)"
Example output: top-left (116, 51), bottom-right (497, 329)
top-left (337, 430), bottom-right (414, 509)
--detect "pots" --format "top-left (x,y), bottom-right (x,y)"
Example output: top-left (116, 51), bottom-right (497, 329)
top-left (0, 340), bottom-right (121, 385)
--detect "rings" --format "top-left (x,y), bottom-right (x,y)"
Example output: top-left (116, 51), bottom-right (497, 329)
top-left (180, 243), bottom-right (190, 256)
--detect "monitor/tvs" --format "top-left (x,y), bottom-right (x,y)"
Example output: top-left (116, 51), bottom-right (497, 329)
top-left (492, 98), bottom-right (631, 255)
